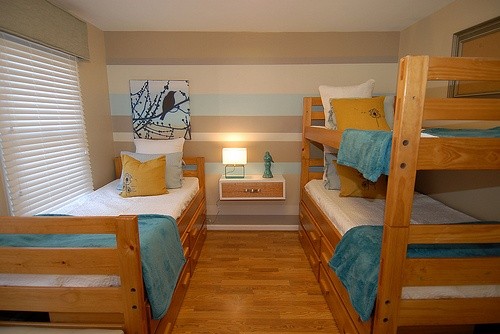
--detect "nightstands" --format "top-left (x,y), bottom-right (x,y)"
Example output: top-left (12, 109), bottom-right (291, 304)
top-left (219, 174), bottom-right (286, 200)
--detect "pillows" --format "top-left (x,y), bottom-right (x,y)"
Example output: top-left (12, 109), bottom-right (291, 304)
top-left (330, 97), bottom-right (390, 132)
top-left (120, 155), bottom-right (169, 197)
top-left (133, 138), bottom-right (185, 180)
top-left (317, 79), bottom-right (375, 128)
top-left (322, 145), bottom-right (340, 190)
top-left (333, 160), bottom-right (388, 199)
top-left (120, 152), bottom-right (182, 188)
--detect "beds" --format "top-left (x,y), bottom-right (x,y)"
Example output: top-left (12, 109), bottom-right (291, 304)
top-left (298, 56), bottom-right (500, 334)
top-left (0, 156), bottom-right (207, 334)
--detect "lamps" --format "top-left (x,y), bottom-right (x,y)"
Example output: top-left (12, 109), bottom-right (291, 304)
top-left (222, 147), bottom-right (248, 178)
